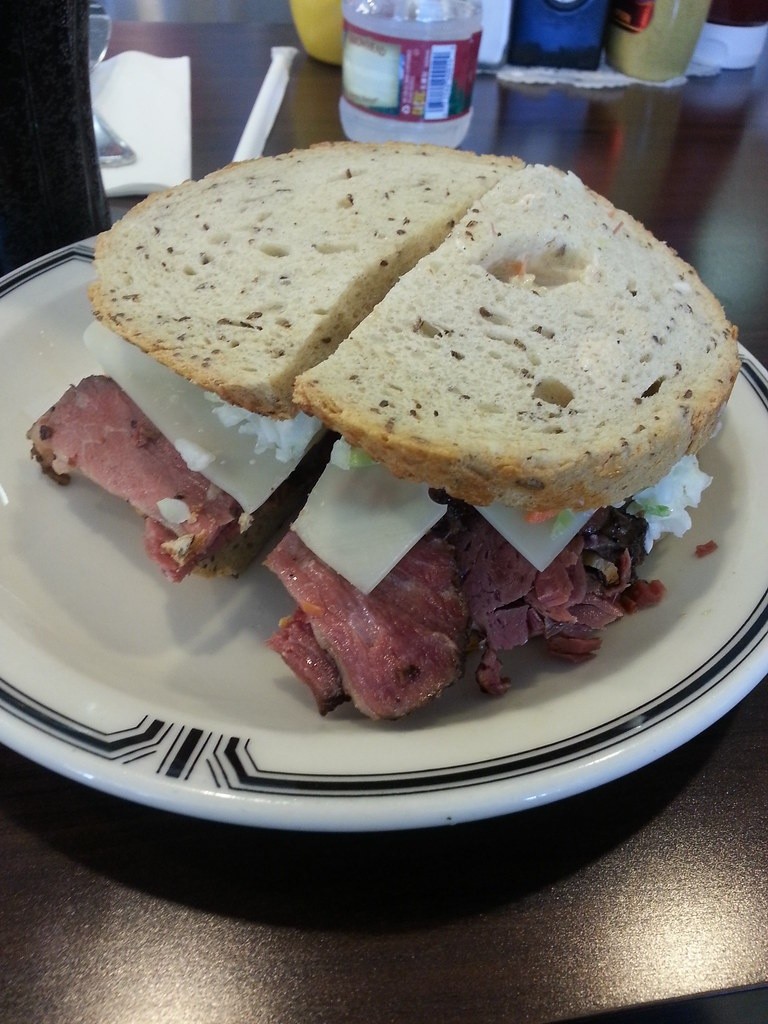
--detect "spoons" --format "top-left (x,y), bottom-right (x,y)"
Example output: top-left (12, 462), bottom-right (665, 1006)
top-left (88, 0), bottom-right (138, 167)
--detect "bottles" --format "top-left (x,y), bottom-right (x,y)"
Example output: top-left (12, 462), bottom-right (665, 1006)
top-left (340, 0), bottom-right (483, 150)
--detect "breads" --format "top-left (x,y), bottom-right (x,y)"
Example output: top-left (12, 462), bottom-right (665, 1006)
top-left (88, 140), bottom-right (526, 418)
top-left (288, 158), bottom-right (740, 513)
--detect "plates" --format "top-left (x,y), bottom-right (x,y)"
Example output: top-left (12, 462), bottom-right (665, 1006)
top-left (0, 237), bottom-right (768, 831)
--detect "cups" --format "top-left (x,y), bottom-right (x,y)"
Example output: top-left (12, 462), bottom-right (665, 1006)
top-left (608, 0), bottom-right (712, 82)
top-left (515, 0), bottom-right (605, 74)
top-left (291, 0), bottom-right (342, 67)
